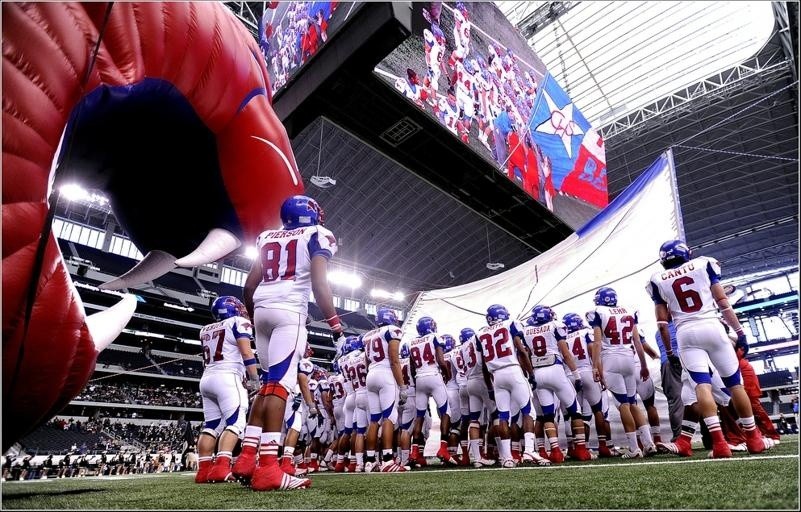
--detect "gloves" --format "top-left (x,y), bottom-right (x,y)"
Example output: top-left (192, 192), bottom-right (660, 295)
top-left (528, 377), bottom-right (538, 390)
top-left (488, 389), bottom-right (495, 400)
top-left (329, 331), bottom-right (347, 364)
top-left (733, 333), bottom-right (750, 359)
top-left (571, 369), bottom-right (583, 393)
top-left (666, 353), bottom-right (682, 378)
top-left (318, 413), bottom-right (326, 428)
top-left (308, 403), bottom-right (317, 420)
top-left (398, 386), bottom-right (409, 405)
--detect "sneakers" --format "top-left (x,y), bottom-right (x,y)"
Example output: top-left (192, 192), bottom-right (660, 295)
top-left (348, 464), bottom-right (357, 472)
top-left (316, 466), bottom-right (329, 472)
top-left (502, 458), bottom-right (520, 468)
top-left (472, 457), bottom-right (497, 467)
top-left (409, 453), bottom-right (425, 465)
top-left (642, 442), bottom-right (658, 457)
top-left (194, 461), bottom-right (213, 483)
top-left (250, 464), bottom-right (311, 491)
top-left (334, 462), bottom-right (345, 471)
top-left (574, 449), bottom-right (598, 460)
top-left (707, 439), bottom-right (733, 457)
top-left (522, 450), bottom-right (552, 466)
top-left (306, 466), bottom-right (315, 474)
top-left (379, 462), bottom-right (407, 472)
top-left (319, 460), bottom-right (336, 471)
top-left (436, 449), bottom-right (458, 466)
top-left (207, 456), bottom-right (236, 483)
top-left (656, 438), bottom-right (692, 457)
top-left (355, 464), bottom-right (365, 472)
top-left (598, 447), bottom-right (619, 457)
top-left (279, 457), bottom-right (307, 475)
top-left (550, 451), bottom-right (565, 464)
top-left (621, 448), bottom-right (643, 459)
top-left (745, 438), bottom-right (766, 454)
top-left (230, 458), bottom-right (258, 480)
top-left (364, 461), bottom-right (380, 472)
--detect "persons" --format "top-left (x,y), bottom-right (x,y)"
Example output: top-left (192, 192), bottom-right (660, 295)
top-left (562, 312), bottom-right (619, 462)
top-left (348, 334), bottom-right (380, 473)
top-left (303, 363), bottom-right (322, 474)
top-left (644, 238), bottom-right (769, 458)
top-left (318, 356), bottom-right (347, 471)
top-left (584, 286), bottom-right (658, 460)
top-left (280, 342), bottom-right (318, 475)
top-left (437, 333), bottom-right (461, 465)
top-left (581, 385), bottom-right (622, 455)
top-left (317, 367), bottom-right (334, 464)
top-left (231, 195), bottom-right (344, 491)
top-left (1, 410), bottom-right (205, 492)
top-left (719, 319), bottom-right (781, 451)
top-left (629, 310), bottom-right (669, 454)
top-left (195, 295), bottom-right (262, 485)
top-left (654, 308), bottom-right (686, 441)
top-left (487, 423), bottom-right (499, 461)
top-left (517, 305), bottom-right (598, 463)
top-left (475, 303), bottom-right (551, 467)
top-left (362, 308), bottom-right (412, 473)
top-left (71, 381), bottom-right (201, 407)
top-left (510, 367), bottom-right (549, 462)
top-left (409, 316), bottom-right (458, 467)
top-left (459, 326), bottom-right (503, 468)
top-left (656, 356), bottom-right (744, 456)
top-left (553, 390), bottom-right (575, 460)
top-left (257, 1), bottom-right (339, 101)
top-left (393, 2), bottom-right (556, 216)
top-left (395, 342), bottom-right (417, 465)
top-left (451, 327), bottom-right (487, 466)
top-left (336, 341), bottom-right (357, 473)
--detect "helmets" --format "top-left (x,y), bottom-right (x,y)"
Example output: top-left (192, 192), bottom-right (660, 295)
top-left (375, 307), bottom-right (399, 328)
top-left (438, 334), bottom-right (456, 353)
top-left (399, 342), bottom-right (411, 359)
top-left (658, 239), bottom-right (693, 270)
top-left (593, 286), bottom-right (618, 307)
top-left (345, 335), bottom-right (364, 354)
top-left (485, 303), bottom-right (510, 326)
top-left (210, 294), bottom-right (249, 322)
top-left (562, 312), bottom-right (584, 333)
top-left (279, 195), bottom-right (327, 230)
top-left (458, 327), bottom-right (476, 344)
top-left (530, 304), bottom-right (557, 326)
top-left (416, 316), bottom-right (438, 337)
